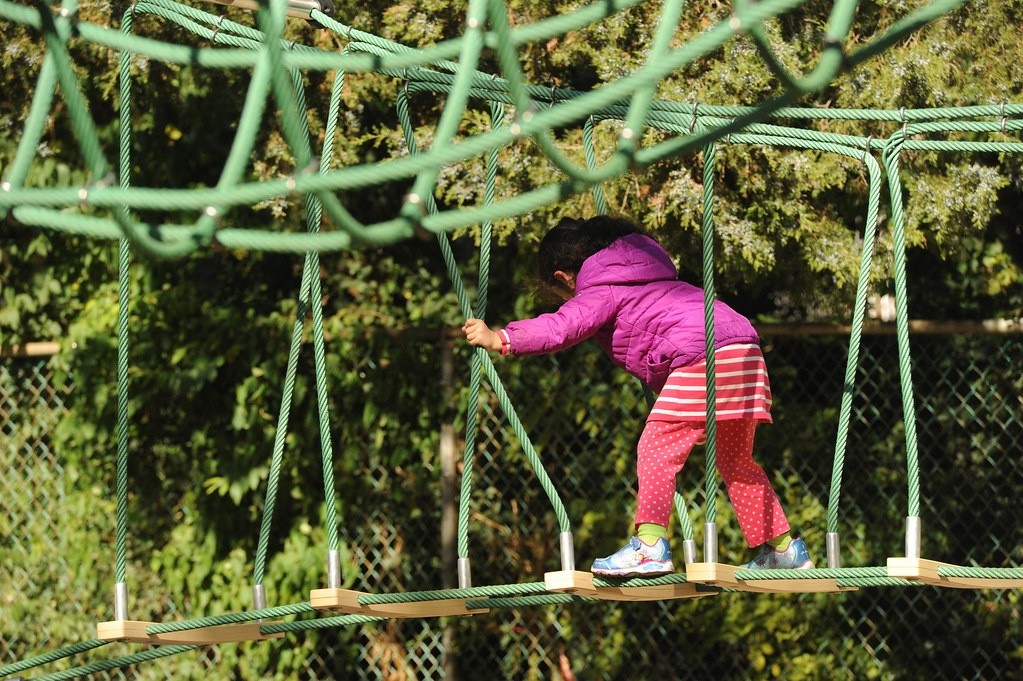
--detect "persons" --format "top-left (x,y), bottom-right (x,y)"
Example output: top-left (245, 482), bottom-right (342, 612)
top-left (462, 215), bottom-right (816, 579)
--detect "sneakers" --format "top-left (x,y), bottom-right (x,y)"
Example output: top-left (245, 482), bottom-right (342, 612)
top-left (737, 537), bottom-right (815, 569)
top-left (591, 537), bottom-right (674, 577)
